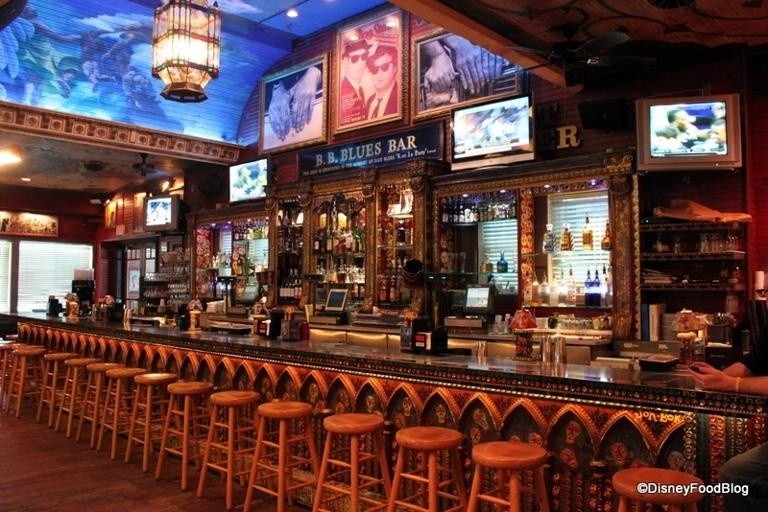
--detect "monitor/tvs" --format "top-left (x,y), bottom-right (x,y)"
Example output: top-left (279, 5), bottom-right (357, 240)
top-left (325, 288), bottom-right (348, 312)
top-left (636, 93), bottom-right (743, 172)
top-left (464, 283), bottom-right (495, 320)
top-left (142, 194), bottom-right (190, 233)
top-left (225, 154), bottom-right (271, 205)
top-left (451, 89), bottom-right (536, 170)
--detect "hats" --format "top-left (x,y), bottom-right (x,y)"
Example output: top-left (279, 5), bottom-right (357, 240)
top-left (342, 40), bottom-right (372, 60)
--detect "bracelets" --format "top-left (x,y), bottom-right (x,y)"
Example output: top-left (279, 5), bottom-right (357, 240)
top-left (735, 376), bottom-right (741, 393)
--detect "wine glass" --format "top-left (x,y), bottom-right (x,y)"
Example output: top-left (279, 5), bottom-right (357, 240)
top-left (698, 231), bottom-right (741, 254)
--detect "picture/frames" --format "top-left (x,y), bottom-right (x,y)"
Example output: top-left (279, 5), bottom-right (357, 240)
top-left (329, 7), bottom-right (410, 143)
top-left (410, 28), bottom-right (526, 127)
top-left (258, 51), bottom-right (330, 158)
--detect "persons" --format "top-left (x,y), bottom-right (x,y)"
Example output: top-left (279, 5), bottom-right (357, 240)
top-left (269, 64), bottom-right (323, 141)
top-left (420, 35), bottom-right (504, 96)
top-left (654, 104), bottom-right (726, 154)
top-left (688, 266), bottom-right (768, 512)
top-left (372, 259), bottom-right (430, 316)
top-left (364, 46), bottom-right (398, 120)
top-left (103, 295), bottom-right (114, 305)
top-left (340, 40), bottom-right (372, 126)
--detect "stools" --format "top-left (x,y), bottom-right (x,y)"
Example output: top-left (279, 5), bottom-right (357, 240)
top-left (386, 426), bottom-right (464, 512)
top-left (312, 412), bottom-right (392, 512)
top-left (196, 390), bottom-right (274, 512)
top-left (612, 468), bottom-right (705, 512)
top-left (465, 441), bottom-right (550, 512)
top-left (243, 400), bottom-right (320, 512)
top-left (0, 339), bottom-right (226, 491)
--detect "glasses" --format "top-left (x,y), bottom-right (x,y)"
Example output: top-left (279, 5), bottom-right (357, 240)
top-left (349, 52), bottom-right (369, 63)
top-left (369, 61), bottom-right (392, 75)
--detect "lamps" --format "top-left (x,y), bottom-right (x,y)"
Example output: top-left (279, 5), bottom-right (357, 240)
top-left (287, 9), bottom-right (297, 17)
top-left (0, 143), bottom-right (23, 165)
top-left (152, 0), bottom-right (222, 103)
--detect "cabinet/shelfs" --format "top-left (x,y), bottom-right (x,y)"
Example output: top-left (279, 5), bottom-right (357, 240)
top-left (636, 171), bottom-right (750, 294)
top-left (274, 179), bottom-right (414, 307)
top-left (434, 165), bottom-right (613, 310)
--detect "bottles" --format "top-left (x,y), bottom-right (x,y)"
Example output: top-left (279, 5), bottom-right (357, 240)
top-left (526, 213), bottom-right (619, 310)
top-left (449, 203), bottom-right (515, 226)
top-left (477, 251), bottom-right (495, 275)
top-left (278, 217), bottom-right (413, 308)
top-left (496, 249), bottom-right (510, 275)
